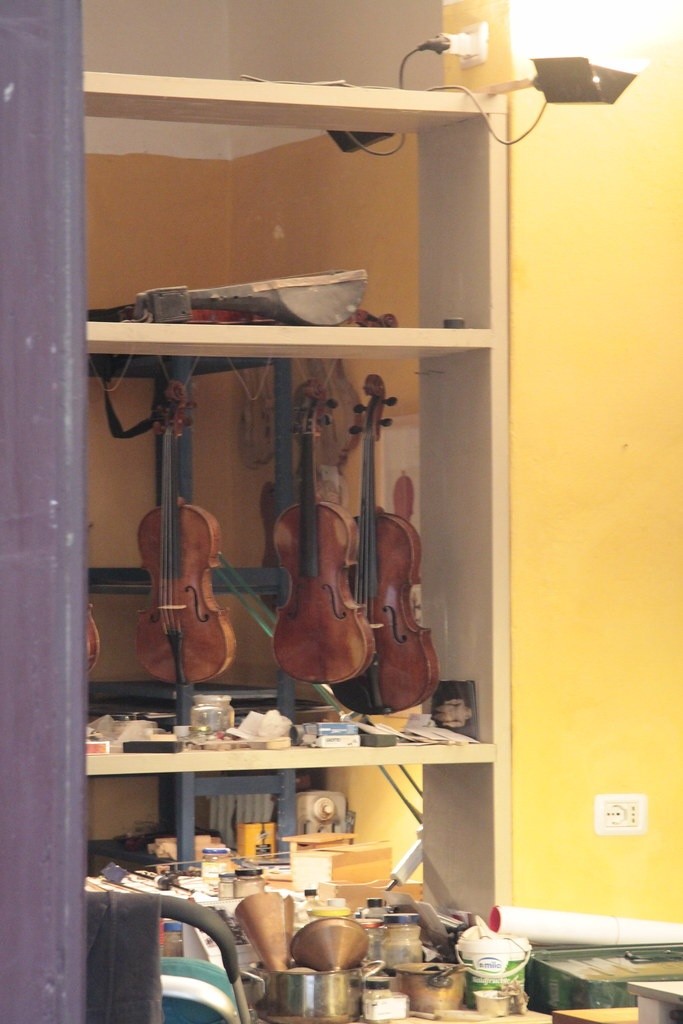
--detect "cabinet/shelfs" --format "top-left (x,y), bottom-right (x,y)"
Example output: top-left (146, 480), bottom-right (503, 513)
top-left (85, 69), bottom-right (511, 922)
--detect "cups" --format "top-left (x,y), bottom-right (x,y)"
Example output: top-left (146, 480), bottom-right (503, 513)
top-left (190, 693), bottom-right (235, 738)
top-left (472, 990), bottom-right (518, 1016)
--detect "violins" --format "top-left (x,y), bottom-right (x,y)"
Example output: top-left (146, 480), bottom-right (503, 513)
top-left (135, 379), bottom-right (237, 687)
top-left (270, 376), bottom-right (378, 687)
top-left (105, 305), bottom-right (399, 329)
top-left (330, 373), bottom-right (440, 714)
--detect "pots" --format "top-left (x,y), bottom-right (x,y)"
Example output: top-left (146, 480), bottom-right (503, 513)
top-left (268, 968), bottom-right (364, 1024)
top-left (394, 960), bottom-right (469, 1013)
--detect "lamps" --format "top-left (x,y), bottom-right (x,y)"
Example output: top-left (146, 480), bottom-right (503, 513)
top-left (484, 56), bottom-right (637, 106)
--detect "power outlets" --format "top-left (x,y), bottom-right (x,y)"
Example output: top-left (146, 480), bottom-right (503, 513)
top-left (460, 22), bottom-right (487, 68)
top-left (594, 794), bottom-right (648, 835)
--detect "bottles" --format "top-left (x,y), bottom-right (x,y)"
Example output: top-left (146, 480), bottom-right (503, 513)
top-left (295, 889), bottom-right (325, 927)
top-left (201, 846), bottom-right (231, 884)
top-left (232, 866), bottom-right (266, 902)
top-left (219, 873), bottom-right (241, 901)
top-left (361, 897), bottom-right (389, 919)
top-left (382, 912), bottom-right (423, 968)
top-left (362, 978), bottom-right (391, 1023)
top-left (355, 916), bottom-right (387, 961)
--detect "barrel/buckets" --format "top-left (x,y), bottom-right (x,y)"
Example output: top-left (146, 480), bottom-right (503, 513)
top-left (454, 935), bottom-right (532, 1011)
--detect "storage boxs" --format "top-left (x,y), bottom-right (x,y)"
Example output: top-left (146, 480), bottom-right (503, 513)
top-left (235, 821), bottom-right (278, 859)
top-left (310, 735), bottom-right (361, 747)
top-left (292, 840), bottom-right (392, 890)
top-left (303, 722), bottom-right (358, 736)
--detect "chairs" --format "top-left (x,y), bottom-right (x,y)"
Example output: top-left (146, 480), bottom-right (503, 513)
top-left (86, 891), bottom-right (251, 1024)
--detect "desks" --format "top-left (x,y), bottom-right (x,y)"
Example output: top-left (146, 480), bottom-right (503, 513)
top-left (256, 1009), bottom-right (553, 1024)
